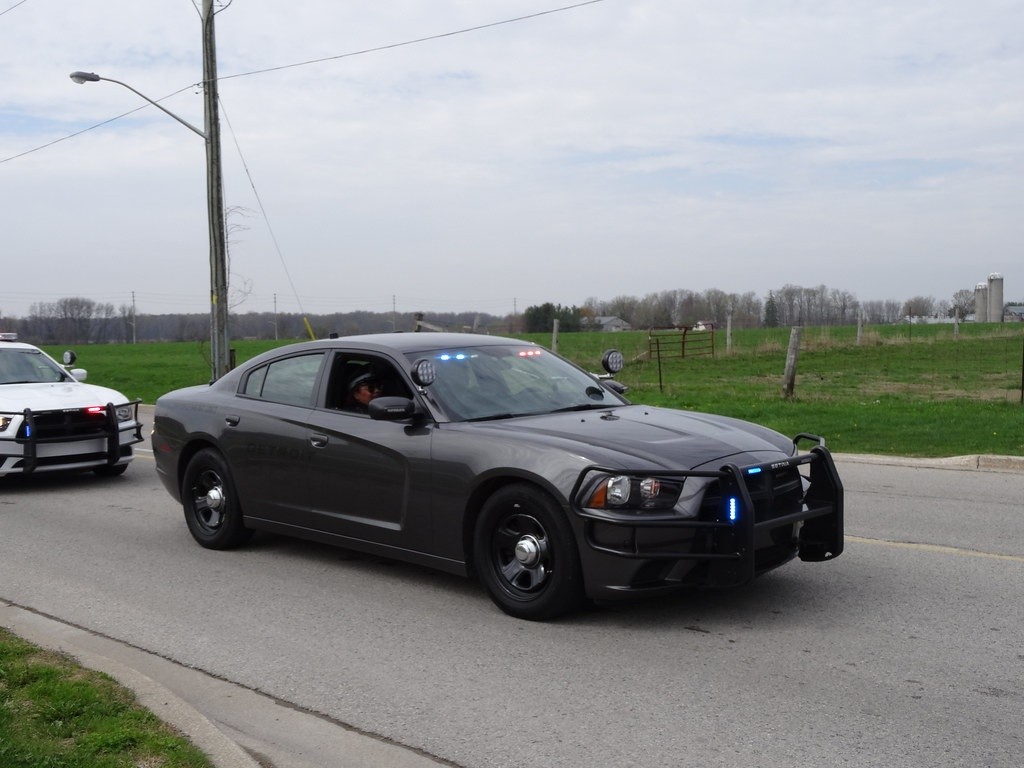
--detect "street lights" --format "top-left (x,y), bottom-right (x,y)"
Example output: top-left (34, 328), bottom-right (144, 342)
top-left (65, 70), bottom-right (232, 382)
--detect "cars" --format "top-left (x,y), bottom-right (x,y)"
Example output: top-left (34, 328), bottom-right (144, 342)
top-left (0, 331), bottom-right (146, 481)
top-left (145, 329), bottom-right (847, 627)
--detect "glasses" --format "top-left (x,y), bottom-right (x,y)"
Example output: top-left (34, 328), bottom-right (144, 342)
top-left (359, 383), bottom-right (381, 393)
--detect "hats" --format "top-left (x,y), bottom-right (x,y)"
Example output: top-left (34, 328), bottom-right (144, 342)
top-left (340, 370), bottom-right (376, 395)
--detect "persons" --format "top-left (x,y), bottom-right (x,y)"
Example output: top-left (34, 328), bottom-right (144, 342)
top-left (445, 365), bottom-right (470, 397)
top-left (335, 364), bottom-right (382, 414)
